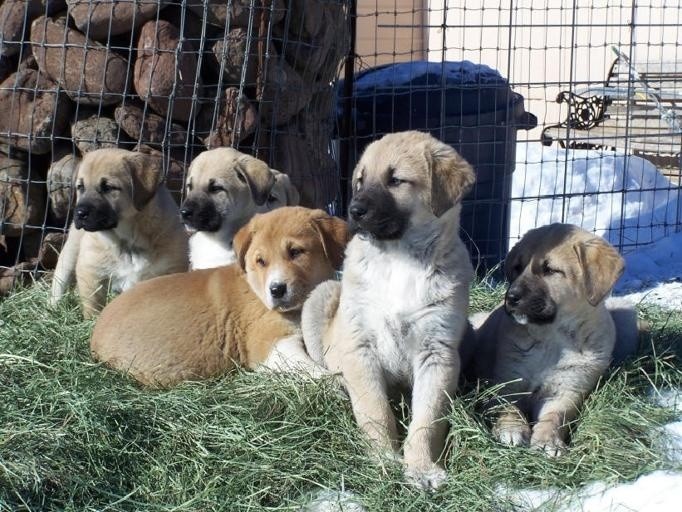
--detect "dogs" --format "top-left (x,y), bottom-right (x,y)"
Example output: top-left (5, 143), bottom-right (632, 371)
top-left (179, 147), bottom-right (300, 270)
top-left (470, 223), bottom-right (626, 459)
top-left (50, 148), bottom-right (189, 321)
top-left (91, 206), bottom-right (354, 400)
top-left (301, 131), bottom-right (476, 494)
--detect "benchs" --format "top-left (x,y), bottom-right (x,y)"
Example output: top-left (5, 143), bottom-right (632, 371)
top-left (541, 58), bottom-right (682, 156)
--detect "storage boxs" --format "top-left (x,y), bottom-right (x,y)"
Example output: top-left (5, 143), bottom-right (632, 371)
top-left (331, 93), bottom-right (537, 285)
